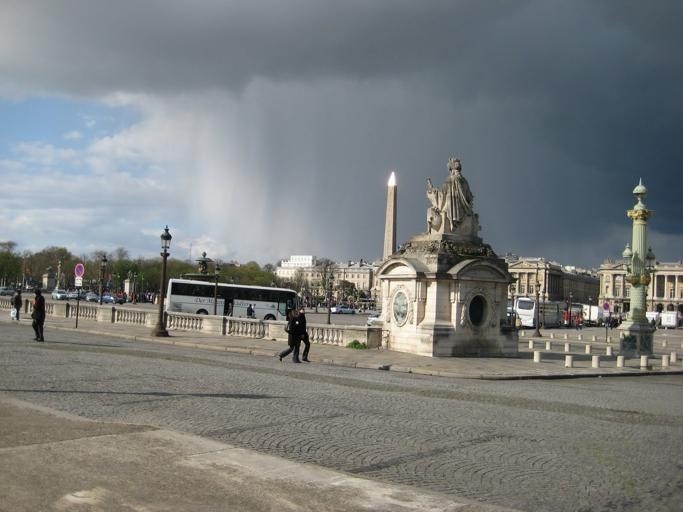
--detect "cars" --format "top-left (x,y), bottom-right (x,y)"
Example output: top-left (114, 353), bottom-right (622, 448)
top-left (330, 304), bottom-right (355, 314)
top-left (52, 288), bottom-right (126, 305)
top-left (0, 286), bottom-right (16, 295)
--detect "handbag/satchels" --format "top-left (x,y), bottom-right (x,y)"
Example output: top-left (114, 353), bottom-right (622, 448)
top-left (285, 322), bottom-right (298, 333)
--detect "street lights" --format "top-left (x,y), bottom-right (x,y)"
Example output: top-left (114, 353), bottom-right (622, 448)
top-left (213, 261), bottom-right (221, 314)
top-left (99, 254), bottom-right (108, 304)
top-left (509, 284), bottom-right (516, 327)
top-left (130, 270), bottom-right (137, 304)
top-left (150, 225), bottom-right (172, 338)
top-left (326, 272), bottom-right (336, 324)
top-left (565, 288), bottom-right (595, 327)
top-left (531, 279), bottom-right (545, 336)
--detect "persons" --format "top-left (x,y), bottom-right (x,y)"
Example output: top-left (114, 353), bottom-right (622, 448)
top-left (298, 305), bottom-right (310, 362)
top-left (30, 288), bottom-right (46, 343)
top-left (515, 315), bottom-right (521, 337)
top-left (420, 159), bottom-right (474, 235)
top-left (226, 303), bottom-right (232, 317)
top-left (562, 307), bottom-right (569, 327)
top-left (12, 288), bottom-right (22, 321)
top-left (246, 303), bottom-right (256, 318)
top-left (278, 309), bottom-right (303, 363)
top-left (575, 314), bottom-right (583, 329)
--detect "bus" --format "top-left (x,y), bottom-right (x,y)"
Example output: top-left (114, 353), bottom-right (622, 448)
top-left (514, 296), bottom-right (567, 328)
top-left (165, 276), bottom-right (298, 321)
top-left (619, 298), bottom-right (679, 327)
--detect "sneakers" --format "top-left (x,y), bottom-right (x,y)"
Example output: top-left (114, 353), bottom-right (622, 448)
top-left (279, 353), bottom-right (310, 363)
